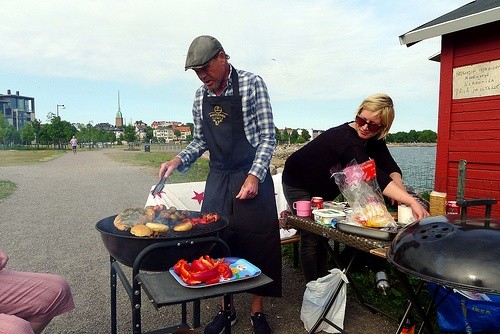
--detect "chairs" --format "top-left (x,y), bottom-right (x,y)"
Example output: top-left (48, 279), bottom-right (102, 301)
top-left (456, 198), bottom-right (496, 219)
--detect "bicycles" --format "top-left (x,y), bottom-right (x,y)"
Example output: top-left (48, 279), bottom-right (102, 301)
top-left (71, 145), bottom-right (78, 159)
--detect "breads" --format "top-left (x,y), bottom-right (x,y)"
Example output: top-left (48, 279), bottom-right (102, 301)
top-left (113, 204), bottom-right (169, 236)
top-left (173, 222), bottom-right (193, 231)
top-left (363, 203), bottom-right (386, 222)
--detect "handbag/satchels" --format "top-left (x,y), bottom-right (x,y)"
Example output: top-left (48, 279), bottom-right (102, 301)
top-left (300, 267), bottom-right (349, 334)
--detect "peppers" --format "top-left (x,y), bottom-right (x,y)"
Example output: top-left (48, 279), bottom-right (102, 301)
top-left (174, 255), bottom-right (233, 285)
top-left (183, 212), bottom-right (220, 225)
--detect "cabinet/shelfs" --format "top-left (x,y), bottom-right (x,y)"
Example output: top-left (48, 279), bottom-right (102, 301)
top-left (110, 238), bottom-right (235, 334)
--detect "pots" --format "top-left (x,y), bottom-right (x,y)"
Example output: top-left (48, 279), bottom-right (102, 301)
top-left (94, 210), bottom-right (229, 272)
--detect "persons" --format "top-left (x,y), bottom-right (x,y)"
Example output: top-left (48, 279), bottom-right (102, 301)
top-left (0, 248), bottom-right (76, 334)
top-left (159, 36), bottom-right (281, 334)
top-left (282, 93), bottom-right (430, 284)
top-left (70, 136), bottom-right (78, 155)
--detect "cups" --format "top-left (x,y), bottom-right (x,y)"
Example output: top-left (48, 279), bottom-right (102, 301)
top-left (293, 201), bottom-right (311, 216)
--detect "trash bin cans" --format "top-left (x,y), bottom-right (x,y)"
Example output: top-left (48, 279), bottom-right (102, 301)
top-left (145, 145), bottom-right (150, 152)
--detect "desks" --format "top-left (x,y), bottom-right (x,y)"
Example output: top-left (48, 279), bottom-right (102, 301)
top-left (282, 210), bottom-right (444, 334)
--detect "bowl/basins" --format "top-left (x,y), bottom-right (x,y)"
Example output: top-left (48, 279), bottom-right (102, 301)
top-left (312, 209), bottom-right (346, 225)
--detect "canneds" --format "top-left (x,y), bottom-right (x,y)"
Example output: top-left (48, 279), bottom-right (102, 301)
top-left (310, 197), bottom-right (323, 219)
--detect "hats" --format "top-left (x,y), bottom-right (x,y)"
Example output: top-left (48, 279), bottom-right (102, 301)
top-left (185, 34), bottom-right (223, 71)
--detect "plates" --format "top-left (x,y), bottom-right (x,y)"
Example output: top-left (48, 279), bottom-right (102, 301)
top-left (169, 257), bottom-right (260, 289)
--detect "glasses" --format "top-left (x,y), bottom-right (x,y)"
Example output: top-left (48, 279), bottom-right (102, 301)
top-left (355, 114), bottom-right (384, 134)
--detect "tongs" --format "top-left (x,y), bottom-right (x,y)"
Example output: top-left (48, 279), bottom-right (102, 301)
top-left (152, 172), bottom-right (166, 196)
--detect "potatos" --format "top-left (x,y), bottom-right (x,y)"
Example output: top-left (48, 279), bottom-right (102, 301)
top-left (161, 207), bottom-right (190, 221)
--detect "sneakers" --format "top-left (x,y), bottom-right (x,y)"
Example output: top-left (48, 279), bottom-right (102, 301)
top-left (248, 311), bottom-right (273, 334)
top-left (205, 308), bottom-right (238, 334)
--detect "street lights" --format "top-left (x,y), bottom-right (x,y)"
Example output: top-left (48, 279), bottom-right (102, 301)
top-left (57, 104), bottom-right (65, 117)
